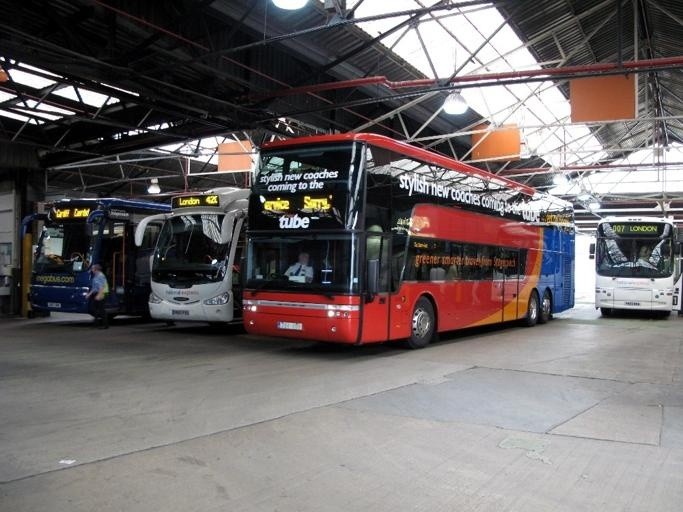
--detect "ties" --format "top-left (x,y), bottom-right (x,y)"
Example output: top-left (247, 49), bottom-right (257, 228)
top-left (295, 265), bottom-right (302, 276)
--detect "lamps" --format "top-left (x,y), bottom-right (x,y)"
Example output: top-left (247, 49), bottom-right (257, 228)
top-left (442, 41), bottom-right (468, 116)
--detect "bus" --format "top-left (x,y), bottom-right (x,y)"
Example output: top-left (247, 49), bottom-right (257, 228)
top-left (241, 133), bottom-right (576, 349)
top-left (132, 185), bottom-right (249, 333)
top-left (18, 196), bottom-right (169, 321)
top-left (586, 214), bottom-right (683, 321)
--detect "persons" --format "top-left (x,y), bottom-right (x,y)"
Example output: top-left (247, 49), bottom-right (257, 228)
top-left (85, 265), bottom-right (109, 329)
top-left (284, 252), bottom-right (314, 284)
